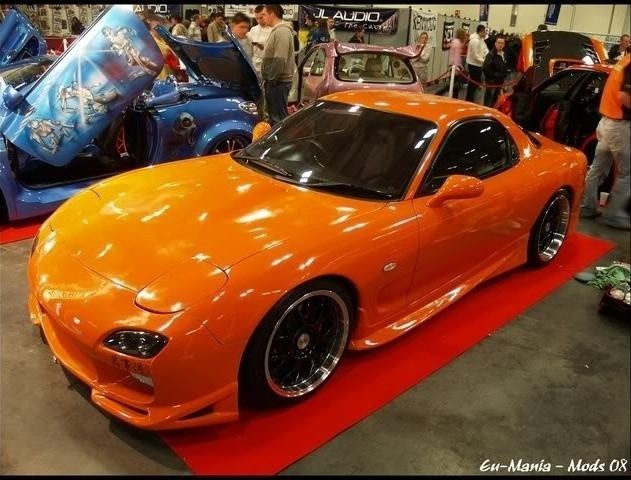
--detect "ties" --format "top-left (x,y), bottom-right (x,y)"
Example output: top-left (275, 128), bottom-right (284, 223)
top-left (186, 9), bottom-right (199, 20)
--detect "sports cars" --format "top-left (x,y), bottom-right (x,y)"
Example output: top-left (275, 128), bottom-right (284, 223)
top-left (0, 2), bottom-right (618, 433)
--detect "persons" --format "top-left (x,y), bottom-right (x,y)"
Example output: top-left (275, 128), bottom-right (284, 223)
top-left (304, 13), bottom-right (336, 46)
top-left (579, 33), bottom-right (631, 230)
top-left (71, 16), bottom-right (86, 35)
top-left (142, 4), bottom-right (298, 129)
top-left (409, 33), bottom-right (431, 93)
top-left (449, 24), bottom-right (509, 108)
top-left (348, 25), bottom-right (365, 65)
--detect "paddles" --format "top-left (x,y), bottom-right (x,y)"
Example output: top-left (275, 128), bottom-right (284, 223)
top-left (1, 217), bottom-right (46, 244)
top-left (168, 231), bottom-right (613, 476)
